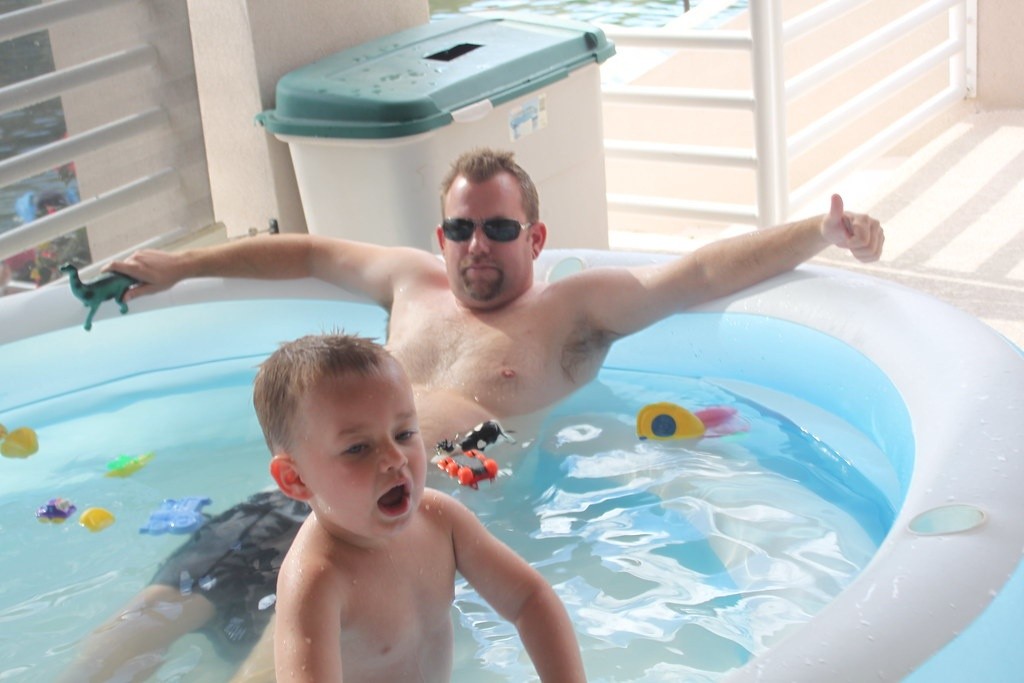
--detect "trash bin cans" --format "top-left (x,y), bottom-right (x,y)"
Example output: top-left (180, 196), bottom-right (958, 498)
top-left (254, 9), bottom-right (617, 252)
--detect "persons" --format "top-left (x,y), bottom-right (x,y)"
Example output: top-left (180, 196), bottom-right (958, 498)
top-left (100, 149), bottom-right (885, 420)
top-left (254, 337), bottom-right (589, 683)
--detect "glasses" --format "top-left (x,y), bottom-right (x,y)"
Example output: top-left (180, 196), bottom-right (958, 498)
top-left (442, 217), bottom-right (531, 241)
